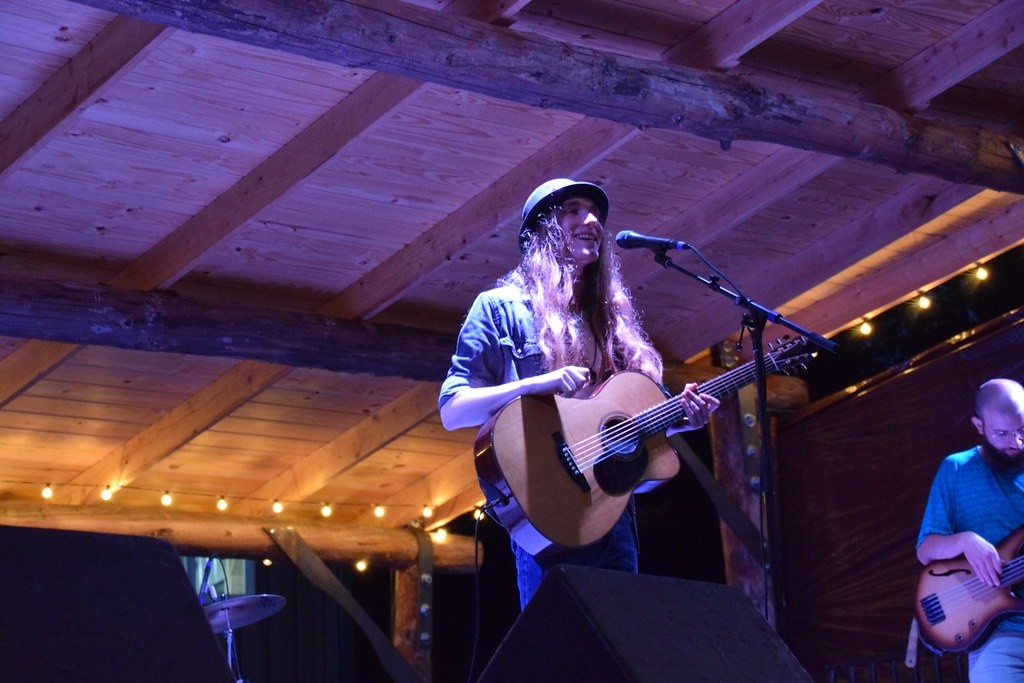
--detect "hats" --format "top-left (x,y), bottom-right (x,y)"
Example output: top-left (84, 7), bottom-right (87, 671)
top-left (518, 178), bottom-right (609, 257)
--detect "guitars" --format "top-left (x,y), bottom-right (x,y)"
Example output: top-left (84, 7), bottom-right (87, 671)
top-left (473, 333), bottom-right (819, 563)
top-left (913, 523), bottom-right (1024, 657)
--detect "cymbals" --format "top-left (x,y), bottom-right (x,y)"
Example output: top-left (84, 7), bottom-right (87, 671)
top-left (202, 593), bottom-right (288, 634)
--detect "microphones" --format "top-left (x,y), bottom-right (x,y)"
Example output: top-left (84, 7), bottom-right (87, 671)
top-left (615, 230), bottom-right (691, 250)
top-left (199, 560), bottom-right (213, 606)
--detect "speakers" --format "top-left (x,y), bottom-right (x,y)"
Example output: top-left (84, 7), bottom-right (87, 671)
top-left (1, 525), bottom-right (238, 682)
top-left (476, 562), bottom-right (812, 683)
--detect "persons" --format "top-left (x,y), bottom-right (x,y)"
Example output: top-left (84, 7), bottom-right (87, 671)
top-left (438, 179), bottom-right (722, 613)
top-left (915, 378), bottom-right (1024, 683)
